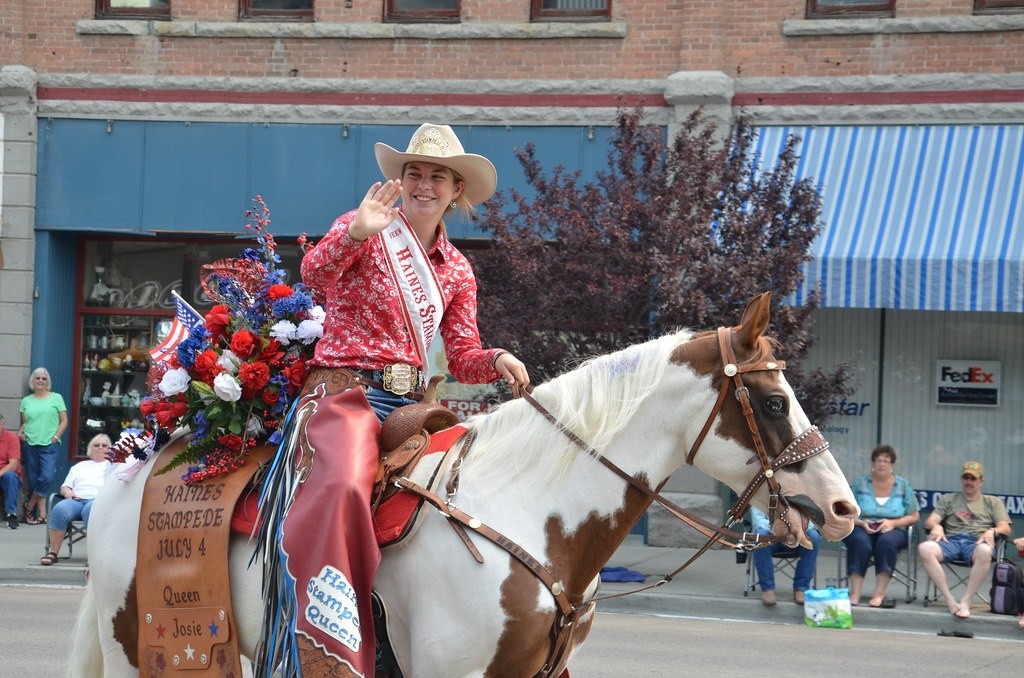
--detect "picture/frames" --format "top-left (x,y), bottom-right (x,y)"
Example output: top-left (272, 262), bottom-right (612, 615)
top-left (934, 357), bottom-right (1002, 408)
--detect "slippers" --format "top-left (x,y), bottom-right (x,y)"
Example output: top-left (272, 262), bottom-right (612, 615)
top-left (882, 595), bottom-right (896, 607)
top-left (937, 627), bottom-right (974, 638)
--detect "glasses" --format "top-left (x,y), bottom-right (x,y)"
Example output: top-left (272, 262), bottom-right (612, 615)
top-left (35, 377), bottom-right (46, 381)
top-left (961, 474), bottom-right (978, 480)
top-left (94, 444), bottom-right (107, 447)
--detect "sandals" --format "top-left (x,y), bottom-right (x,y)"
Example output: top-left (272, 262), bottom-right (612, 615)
top-left (39, 551), bottom-right (58, 565)
top-left (30, 515), bottom-right (46, 524)
top-left (23, 501), bottom-right (35, 525)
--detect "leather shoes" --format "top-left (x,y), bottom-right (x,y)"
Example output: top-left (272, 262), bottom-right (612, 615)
top-left (761, 589), bottom-right (776, 606)
top-left (793, 586), bottom-right (806, 605)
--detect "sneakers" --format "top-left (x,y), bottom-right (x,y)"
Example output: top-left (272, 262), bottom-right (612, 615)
top-left (6, 513), bottom-right (21, 530)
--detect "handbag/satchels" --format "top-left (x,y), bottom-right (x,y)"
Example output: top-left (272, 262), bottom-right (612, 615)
top-left (803, 586), bottom-right (854, 629)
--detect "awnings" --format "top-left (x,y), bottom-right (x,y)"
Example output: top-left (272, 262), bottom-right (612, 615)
top-left (702, 120), bottom-right (1024, 312)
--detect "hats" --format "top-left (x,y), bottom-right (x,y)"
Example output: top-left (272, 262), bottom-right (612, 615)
top-left (372, 123), bottom-right (498, 210)
top-left (959, 461), bottom-right (985, 479)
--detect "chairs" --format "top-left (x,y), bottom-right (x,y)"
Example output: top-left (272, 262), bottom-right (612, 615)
top-left (738, 510), bottom-right (820, 597)
top-left (837, 490), bottom-right (920, 605)
top-left (43, 465), bottom-right (97, 563)
top-left (922, 526), bottom-right (1018, 608)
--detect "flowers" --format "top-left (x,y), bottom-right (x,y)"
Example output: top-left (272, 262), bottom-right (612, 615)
top-left (104, 187), bottom-right (328, 486)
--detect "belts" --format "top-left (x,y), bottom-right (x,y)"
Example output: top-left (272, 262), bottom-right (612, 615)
top-left (342, 362), bottom-right (427, 396)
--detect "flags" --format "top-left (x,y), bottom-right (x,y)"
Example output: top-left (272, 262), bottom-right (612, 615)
top-left (148, 296), bottom-right (205, 370)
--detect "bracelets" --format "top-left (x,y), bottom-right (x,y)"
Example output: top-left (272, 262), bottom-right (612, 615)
top-left (492, 351), bottom-right (514, 368)
top-left (54, 435), bottom-right (59, 440)
top-left (989, 527), bottom-right (996, 536)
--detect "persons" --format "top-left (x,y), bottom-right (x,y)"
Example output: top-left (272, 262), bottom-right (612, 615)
top-left (18, 367), bottom-right (67, 526)
top-left (277, 122), bottom-right (528, 677)
top-left (1014, 535), bottom-right (1024, 627)
top-left (0, 414), bottom-right (24, 529)
top-left (918, 460), bottom-right (1013, 618)
top-left (842, 444), bottom-right (922, 605)
top-left (39, 432), bottom-right (114, 566)
top-left (749, 504), bottom-right (821, 605)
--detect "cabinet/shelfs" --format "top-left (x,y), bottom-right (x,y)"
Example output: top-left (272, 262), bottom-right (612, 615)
top-left (70, 234), bottom-right (217, 460)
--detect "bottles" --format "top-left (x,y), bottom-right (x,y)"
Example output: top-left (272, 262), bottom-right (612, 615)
top-left (90, 336), bottom-right (97, 349)
top-left (101, 335), bottom-right (108, 349)
top-left (83, 353), bottom-right (99, 371)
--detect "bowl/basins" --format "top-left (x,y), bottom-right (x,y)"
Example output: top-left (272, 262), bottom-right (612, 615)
top-left (88, 397), bottom-right (103, 405)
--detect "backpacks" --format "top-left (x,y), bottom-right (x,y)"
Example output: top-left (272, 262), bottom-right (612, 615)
top-left (989, 557), bottom-right (1024, 615)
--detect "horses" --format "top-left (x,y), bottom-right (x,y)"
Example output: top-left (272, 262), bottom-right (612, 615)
top-left (64, 292), bottom-right (860, 678)
